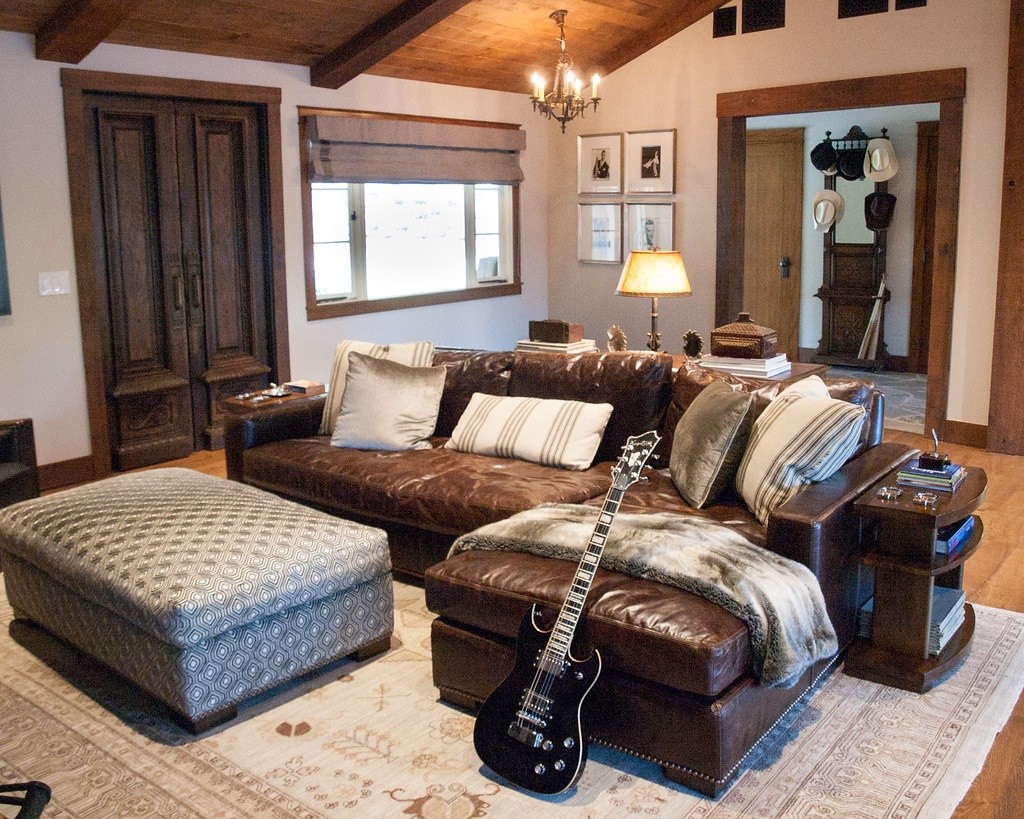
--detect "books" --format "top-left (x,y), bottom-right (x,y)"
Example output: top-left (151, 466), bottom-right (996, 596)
top-left (516, 337), bottom-right (599, 354)
top-left (858, 459), bottom-right (975, 654)
top-left (699, 353), bottom-right (792, 378)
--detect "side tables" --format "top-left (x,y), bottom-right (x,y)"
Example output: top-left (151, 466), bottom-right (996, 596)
top-left (218, 388), bottom-right (311, 411)
top-left (845, 464), bottom-right (987, 694)
top-left (517, 339), bottom-right (598, 353)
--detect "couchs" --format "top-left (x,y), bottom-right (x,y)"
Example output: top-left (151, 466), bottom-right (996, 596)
top-left (226, 349), bottom-right (888, 794)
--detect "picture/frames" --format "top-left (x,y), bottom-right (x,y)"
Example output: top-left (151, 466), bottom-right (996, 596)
top-left (622, 127), bottom-right (678, 197)
top-left (576, 201), bottom-right (623, 266)
top-left (622, 201), bottom-right (676, 265)
top-left (576, 131), bottom-right (623, 196)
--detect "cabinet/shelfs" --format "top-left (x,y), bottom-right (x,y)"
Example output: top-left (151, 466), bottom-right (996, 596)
top-left (0, 418), bottom-right (40, 509)
top-left (669, 353), bottom-right (833, 385)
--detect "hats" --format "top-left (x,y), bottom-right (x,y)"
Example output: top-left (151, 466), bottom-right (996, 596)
top-left (813, 189), bottom-right (846, 233)
top-left (863, 139), bottom-right (899, 183)
top-left (864, 192), bottom-right (897, 232)
top-left (810, 142), bottom-right (838, 176)
top-left (837, 149), bottom-right (867, 182)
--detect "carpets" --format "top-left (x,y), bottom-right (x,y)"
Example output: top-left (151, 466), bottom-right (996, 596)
top-left (0, 573), bottom-right (1024, 819)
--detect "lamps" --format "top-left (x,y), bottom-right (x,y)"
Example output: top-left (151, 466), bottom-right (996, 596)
top-left (613, 250), bottom-right (694, 354)
top-left (528, 9), bottom-right (603, 135)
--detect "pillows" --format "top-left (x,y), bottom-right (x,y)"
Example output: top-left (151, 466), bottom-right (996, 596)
top-left (735, 374), bottom-right (868, 526)
top-left (668, 378), bottom-right (758, 506)
top-left (317, 340), bottom-right (434, 433)
top-left (442, 390), bottom-right (615, 471)
top-left (330, 351), bottom-right (448, 451)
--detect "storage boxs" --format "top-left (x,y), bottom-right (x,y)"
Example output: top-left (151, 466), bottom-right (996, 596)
top-left (709, 311), bottom-right (778, 359)
top-left (527, 320), bottom-right (586, 344)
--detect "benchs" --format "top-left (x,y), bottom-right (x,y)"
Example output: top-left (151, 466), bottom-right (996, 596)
top-left (0, 464), bottom-right (396, 733)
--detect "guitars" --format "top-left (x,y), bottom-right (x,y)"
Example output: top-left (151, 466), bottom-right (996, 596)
top-left (473, 431), bottom-right (664, 795)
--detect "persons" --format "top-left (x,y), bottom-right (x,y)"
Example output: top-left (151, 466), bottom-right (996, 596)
top-left (593, 150), bottom-right (659, 250)
